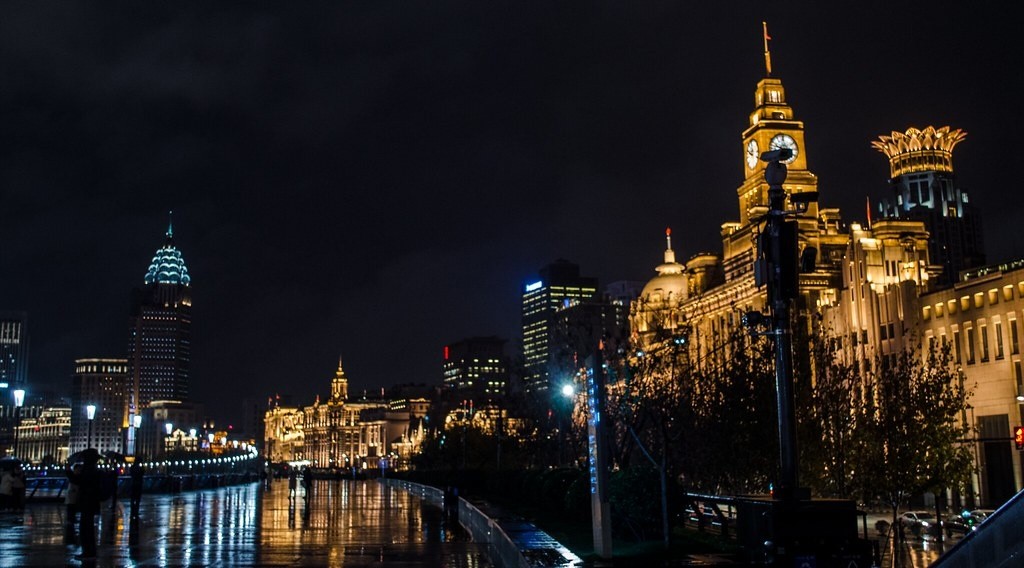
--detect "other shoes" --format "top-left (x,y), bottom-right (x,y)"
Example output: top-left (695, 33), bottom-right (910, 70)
top-left (288, 496), bottom-right (291, 499)
top-left (68, 518), bottom-right (79, 523)
top-left (293, 496), bottom-right (295, 499)
top-left (75, 553), bottom-right (97, 560)
top-left (303, 497), bottom-right (306, 499)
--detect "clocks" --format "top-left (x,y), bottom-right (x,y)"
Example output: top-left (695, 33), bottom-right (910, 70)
top-left (747, 139), bottom-right (759, 169)
top-left (770, 133), bottom-right (798, 165)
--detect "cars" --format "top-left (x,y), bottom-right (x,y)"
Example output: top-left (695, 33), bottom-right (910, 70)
top-left (944, 514), bottom-right (981, 538)
top-left (970, 509), bottom-right (996, 523)
top-left (897, 510), bottom-right (943, 530)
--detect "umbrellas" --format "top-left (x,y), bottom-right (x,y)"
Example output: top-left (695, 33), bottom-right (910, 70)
top-left (67, 449), bottom-right (128, 463)
top-left (0, 456), bottom-right (24, 466)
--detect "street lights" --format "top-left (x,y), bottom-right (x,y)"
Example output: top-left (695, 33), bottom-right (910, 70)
top-left (87, 405), bottom-right (96, 449)
top-left (13, 389), bottom-right (26, 457)
top-left (190, 429), bottom-right (197, 452)
top-left (166, 424), bottom-right (173, 456)
top-left (208, 434), bottom-right (214, 459)
top-left (133, 415), bottom-right (142, 456)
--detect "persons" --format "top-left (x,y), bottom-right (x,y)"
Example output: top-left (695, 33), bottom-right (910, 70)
top-left (0, 463), bottom-right (27, 515)
top-left (303, 466), bottom-right (312, 499)
top-left (64, 454), bottom-right (146, 521)
top-left (289, 468), bottom-right (298, 499)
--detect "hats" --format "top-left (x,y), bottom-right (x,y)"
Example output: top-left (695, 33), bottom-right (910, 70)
top-left (81, 449), bottom-right (101, 461)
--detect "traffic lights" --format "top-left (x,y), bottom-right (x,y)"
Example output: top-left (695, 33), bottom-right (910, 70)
top-left (1014, 426), bottom-right (1024, 451)
